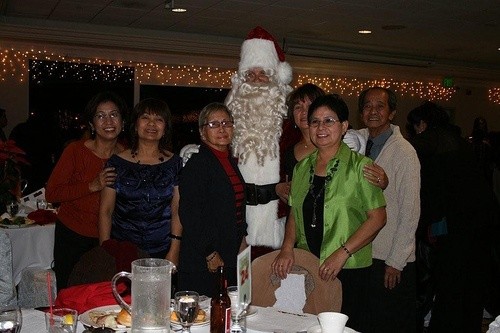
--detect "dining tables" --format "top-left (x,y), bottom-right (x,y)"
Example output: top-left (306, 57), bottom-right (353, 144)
top-left (75, 298), bottom-right (359, 333)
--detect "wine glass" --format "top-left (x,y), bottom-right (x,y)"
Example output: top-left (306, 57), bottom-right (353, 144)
top-left (174, 291), bottom-right (200, 333)
top-left (227, 286), bottom-right (248, 333)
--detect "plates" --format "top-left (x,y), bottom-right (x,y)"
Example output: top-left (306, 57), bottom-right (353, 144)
top-left (246, 305), bottom-right (258, 318)
top-left (79, 304), bottom-right (131, 333)
top-left (0, 219), bottom-right (35, 229)
top-left (307, 325), bottom-right (357, 333)
top-left (170, 308), bottom-right (209, 327)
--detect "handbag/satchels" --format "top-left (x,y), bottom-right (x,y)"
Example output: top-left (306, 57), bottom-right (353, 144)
top-left (27, 200), bottom-right (57, 224)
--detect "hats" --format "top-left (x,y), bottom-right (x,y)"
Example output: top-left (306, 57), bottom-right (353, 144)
top-left (239, 26), bottom-right (292, 85)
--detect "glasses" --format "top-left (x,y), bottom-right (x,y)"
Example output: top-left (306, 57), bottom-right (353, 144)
top-left (203, 121), bottom-right (233, 128)
top-left (307, 117), bottom-right (340, 127)
top-left (93, 111), bottom-right (120, 121)
top-left (239, 70), bottom-right (269, 82)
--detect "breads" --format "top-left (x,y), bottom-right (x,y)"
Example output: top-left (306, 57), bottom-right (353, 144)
top-left (116, 308), bottom-right (132, 327)
top-left (170, 308), bottom-right (206, 324)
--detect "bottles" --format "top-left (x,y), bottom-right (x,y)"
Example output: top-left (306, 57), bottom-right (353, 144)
top-left (210, 266), bottom-right (231, 333)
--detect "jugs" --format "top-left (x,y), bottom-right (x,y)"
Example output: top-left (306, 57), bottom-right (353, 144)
top-left (112, 258), bottom-right (176, 333)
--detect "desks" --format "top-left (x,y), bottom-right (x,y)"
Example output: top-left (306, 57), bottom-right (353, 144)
top-left (4, 223), bottom-right (55, 286)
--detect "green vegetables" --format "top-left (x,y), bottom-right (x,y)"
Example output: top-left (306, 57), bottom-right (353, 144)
top-left (0, 216), bottom-right (26, 228)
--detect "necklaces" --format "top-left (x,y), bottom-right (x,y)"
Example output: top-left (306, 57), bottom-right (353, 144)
top-left (309, 161), bottom-right (341, 228)
top-left (304, 140), bottom-right (308, 148)
top-left (131, 148), bottom-right (164, 165)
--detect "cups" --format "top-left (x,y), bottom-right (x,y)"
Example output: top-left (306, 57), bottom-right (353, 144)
top-left (0, 305), bottom-right (22, 333)
top-left (6, 201), bottom-right (19, 216)
top-left (36, 199), bottom-right (48, 210)
top-left (317, 312), bottom-right (349, 333)
top-left (45, 308), bottom-right (78, 333)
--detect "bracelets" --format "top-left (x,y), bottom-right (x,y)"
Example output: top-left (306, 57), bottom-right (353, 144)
top-left (170, 233), bottom-right (183, 242)
top-left (341, 245), bottom-right (352, 257)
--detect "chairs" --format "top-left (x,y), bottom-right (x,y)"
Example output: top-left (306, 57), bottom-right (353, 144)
top-left (251, 248), bottom-right (342, 315)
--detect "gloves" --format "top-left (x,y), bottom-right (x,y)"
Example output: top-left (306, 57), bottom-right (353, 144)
top-left (181, 145), bottom-right (200, 167)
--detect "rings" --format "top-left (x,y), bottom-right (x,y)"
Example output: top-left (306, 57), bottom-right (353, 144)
top-left (324, 268), bottom-right (329, 271)
top-left (279, 266), bottom-right (282, 267)
top-left (377, 178), bottom-right (380, 183)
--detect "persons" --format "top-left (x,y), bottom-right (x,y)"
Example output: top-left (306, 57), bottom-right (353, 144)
top-left (0, 84), bottom-right (500, 333)
top-left (181, 27), bottom-right (367, 264)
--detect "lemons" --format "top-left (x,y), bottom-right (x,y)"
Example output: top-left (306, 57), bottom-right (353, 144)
top-left (63, 314), bottom-right (73, 324)
